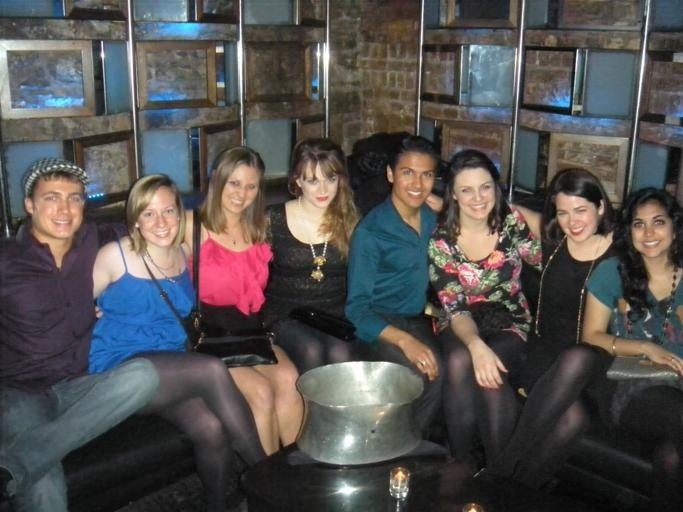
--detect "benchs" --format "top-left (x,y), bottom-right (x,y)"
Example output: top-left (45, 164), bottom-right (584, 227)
top-left (1, 412), bottom-right (199, 512)
top-left (572, 420), bottom-right (661, 509)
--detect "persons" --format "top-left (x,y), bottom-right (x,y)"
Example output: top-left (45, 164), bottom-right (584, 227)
top-left (0, 158), bottom-right (160, 512)
top-left (344, 134), bottom-right (445, 440)
top-left (258, 137), bottom-right (367, 378)
top-left (424, 167), bottom-right (619, 493)
top-left (87, 174), bottom-right (288, 512)
top-left (581, 186), bottom-right (683, 512)
top-left (92, 146), bottom-right (304, 457)
top-left (427, 149), bottom-right (542, 476)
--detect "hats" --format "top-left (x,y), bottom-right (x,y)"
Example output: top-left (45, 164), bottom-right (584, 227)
top-left (21, 156), bottom-right (89, 199)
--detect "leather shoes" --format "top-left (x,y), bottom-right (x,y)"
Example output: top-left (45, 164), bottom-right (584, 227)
top-left (239, 460), bottom-right (320, 512)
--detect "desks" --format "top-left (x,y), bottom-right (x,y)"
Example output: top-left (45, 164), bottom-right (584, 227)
top-left (242, 436), bottom-right (471, 511)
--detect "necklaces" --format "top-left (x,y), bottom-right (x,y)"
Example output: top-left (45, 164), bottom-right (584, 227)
top-left (535, 234), bottom-right (601, 344)
top-left (297, 196), bottom-right (328, 282)
top-left (145, 250), bottom-right (183, 284)
top-left (226, 231), bottom-right (241, 247)
top-left (628, 264), bottom-right (679, 345)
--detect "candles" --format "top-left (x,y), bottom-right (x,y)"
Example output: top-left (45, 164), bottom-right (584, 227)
top-left (387, 463), bottom-right (412, 499)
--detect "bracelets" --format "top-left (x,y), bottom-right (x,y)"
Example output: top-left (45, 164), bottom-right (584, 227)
top-left (612, 336), bottom-right (620, 357)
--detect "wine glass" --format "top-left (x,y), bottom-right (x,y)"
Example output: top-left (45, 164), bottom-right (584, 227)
top-left (389, 466), bottom-right (408, 512)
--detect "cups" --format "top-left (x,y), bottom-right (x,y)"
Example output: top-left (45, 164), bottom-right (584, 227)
top-left (462, 503), bottom-right (484, 512)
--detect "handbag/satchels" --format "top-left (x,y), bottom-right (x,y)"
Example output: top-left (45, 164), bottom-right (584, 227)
top-left (288, 305), bottom-right (358, 344)
top-left (182, 304), bottom-right (278, 369)
top-left (606, 353), bottom-right (682, 388)
top-left (464, 300), bottom-right (514, 337)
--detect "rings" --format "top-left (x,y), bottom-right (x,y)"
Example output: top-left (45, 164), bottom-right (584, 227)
top-left (420, 360), bottom-right (426, 366)
